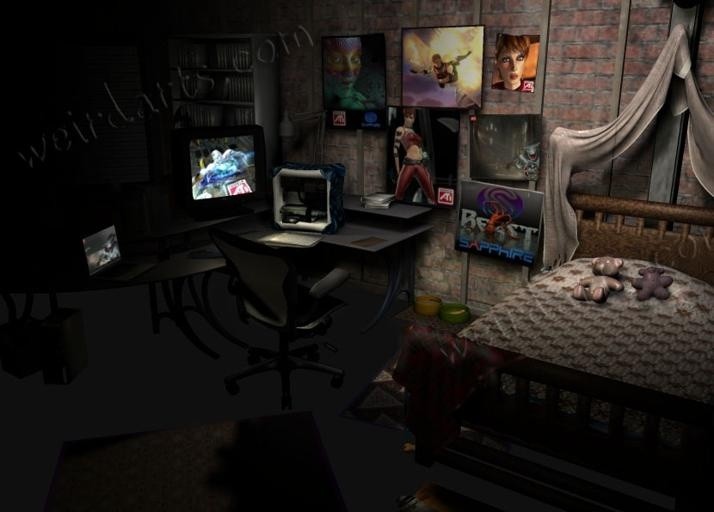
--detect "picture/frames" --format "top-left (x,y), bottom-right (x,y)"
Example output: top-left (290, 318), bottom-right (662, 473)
top-left (318, 31), bottom-right (389, 132)
top-left (77, 221), bottom-right (122, 280)
top-left (399, 24), bottom-right (486, 112)
top-left (384, 105), bottom-right (461, 210)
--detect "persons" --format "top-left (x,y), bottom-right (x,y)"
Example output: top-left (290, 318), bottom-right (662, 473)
top-left (323, 37), bottom-right (371, 110)
top-left (393, 108), bottom-right (438, 204)
top-left (492, 33), bottom-right (535, 93)
top-left (199, 149), bottom-right (248, 177)
top-left (631, 267), bottom-right (673, 300)
top-left (410, 51), bottom-right (472, 88)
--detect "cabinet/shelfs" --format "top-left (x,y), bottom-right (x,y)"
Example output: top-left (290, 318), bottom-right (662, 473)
top-left (137, 28), bottom-right (281, 192)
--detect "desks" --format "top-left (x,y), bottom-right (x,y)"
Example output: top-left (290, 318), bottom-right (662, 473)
top-left (194, 184), bottom-right (437, 337)
top-left (0, 239), bottom-right (248, 368)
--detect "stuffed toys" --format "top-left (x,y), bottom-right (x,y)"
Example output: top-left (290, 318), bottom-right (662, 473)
top-left (573, 256), bottom-right (624, 302)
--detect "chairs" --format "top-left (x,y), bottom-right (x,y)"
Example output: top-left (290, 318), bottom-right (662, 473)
top-left (203, 225), bottom-right (352, 411)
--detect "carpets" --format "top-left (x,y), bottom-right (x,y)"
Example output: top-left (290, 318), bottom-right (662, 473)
top-left (334, 360), bottom-right (411, 432)
top-left (44, 411), bottom-right (346, 512)
top-left (389, 303), bottom-right (478, 334)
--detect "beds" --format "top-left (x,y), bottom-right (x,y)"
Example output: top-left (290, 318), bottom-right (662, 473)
top-left (391, 182), bottom-right (713, 512)
